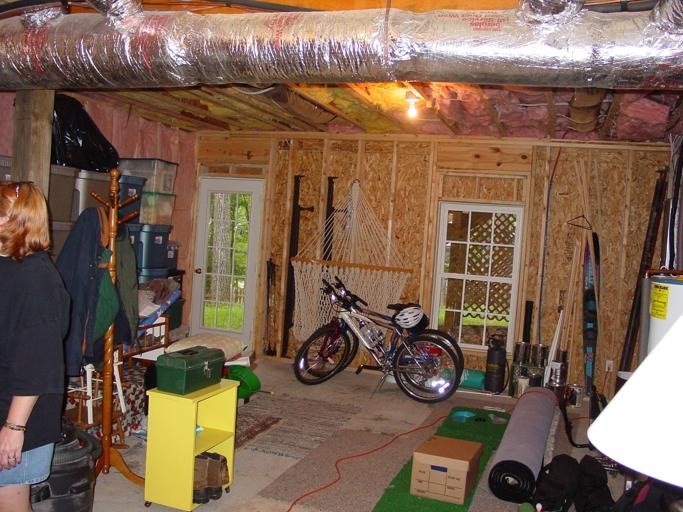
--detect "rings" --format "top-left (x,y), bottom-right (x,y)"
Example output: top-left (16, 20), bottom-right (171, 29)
top-left (7, 457), bottom-right (17, 461)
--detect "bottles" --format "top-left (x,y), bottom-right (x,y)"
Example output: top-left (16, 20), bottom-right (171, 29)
top-left (359, 320), bottom-right (385, 348)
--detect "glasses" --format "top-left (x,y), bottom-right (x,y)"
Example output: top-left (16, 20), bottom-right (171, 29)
top-left (10, 177), bottom-right (37, 201)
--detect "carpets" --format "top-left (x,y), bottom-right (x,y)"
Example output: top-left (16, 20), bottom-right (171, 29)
top-left (372, 407), bottom-right (511, 512)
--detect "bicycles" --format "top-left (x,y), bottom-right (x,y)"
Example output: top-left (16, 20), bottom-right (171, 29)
top-left (293, 275), bottom-right (464, 404)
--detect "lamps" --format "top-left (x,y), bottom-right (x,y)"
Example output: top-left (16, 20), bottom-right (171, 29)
top-left (404, 91), bottom-right (422, 120)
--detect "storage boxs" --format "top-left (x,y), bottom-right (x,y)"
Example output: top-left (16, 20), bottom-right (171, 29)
top-left (410, 435), bottom-right (483, 505)
top-left (0, 157), bottom-right (191, 342)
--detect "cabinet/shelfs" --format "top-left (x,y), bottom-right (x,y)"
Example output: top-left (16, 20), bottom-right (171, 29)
top-left (145, 379), bottom-right (241, 512)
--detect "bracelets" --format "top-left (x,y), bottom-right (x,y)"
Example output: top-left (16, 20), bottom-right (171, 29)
top-left (1, 419), bottom-right (27, 432)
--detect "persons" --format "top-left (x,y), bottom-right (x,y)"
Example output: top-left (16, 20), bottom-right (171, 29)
top-left (0, 177), bottom-right (72, 510)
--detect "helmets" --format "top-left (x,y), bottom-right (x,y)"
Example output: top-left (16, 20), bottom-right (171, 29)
top-left (394, 306), bottom-right (424, 332)
